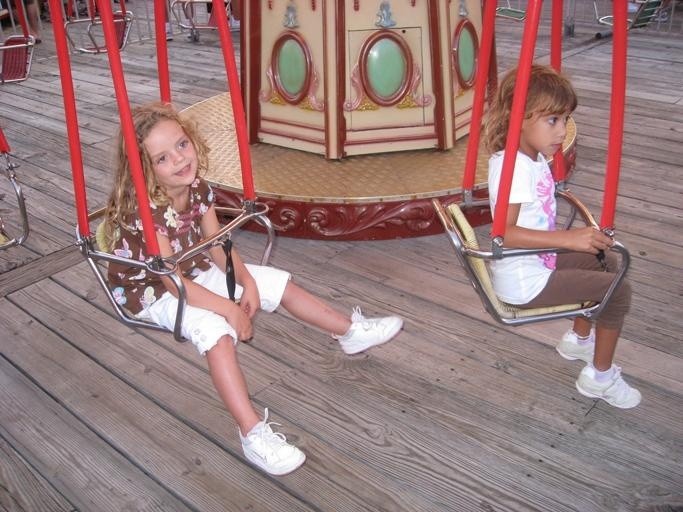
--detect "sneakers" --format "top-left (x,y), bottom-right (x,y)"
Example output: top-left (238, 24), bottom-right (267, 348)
top-left (556, 327), bottom-right (595, 363)
top-left (332, 306), bottom-right (403, 354)
top-left (575, 361), bottom-right (641, 409)
top-left (238, 408), bottom-right (306, 475)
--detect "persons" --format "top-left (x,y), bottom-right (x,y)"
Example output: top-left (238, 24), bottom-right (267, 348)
top-left (479, 64), bottom-right (642, 409)
top-left (96, 102), bottom-right (404, 475)
top-left (650, 0), bottom-right (683, 24)
top-left (0, 0), bottom-right (240, 45)
